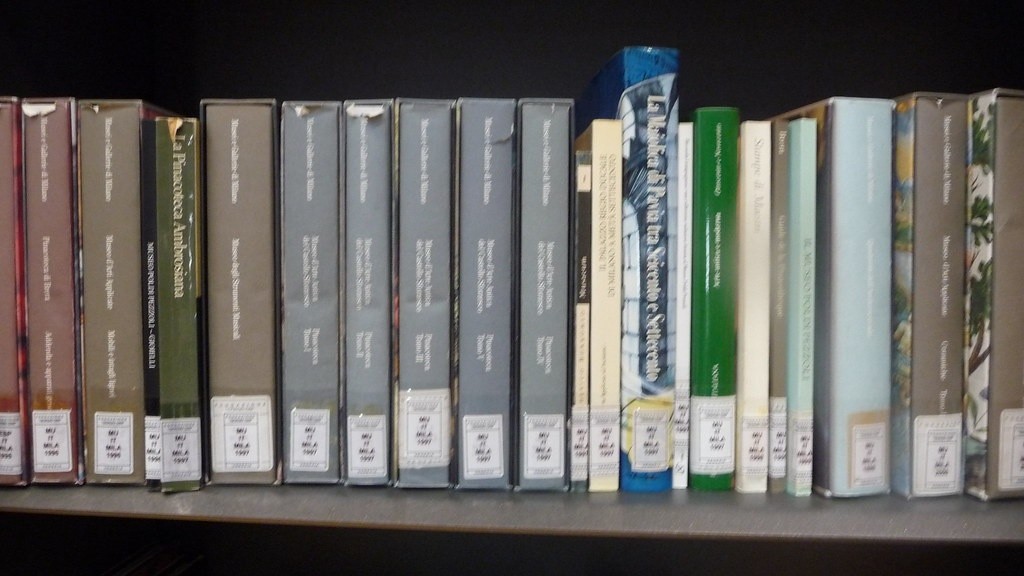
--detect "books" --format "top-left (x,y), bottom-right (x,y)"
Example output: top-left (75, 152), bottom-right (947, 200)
top-left (0, 47), bottom-right (1024, 504)
